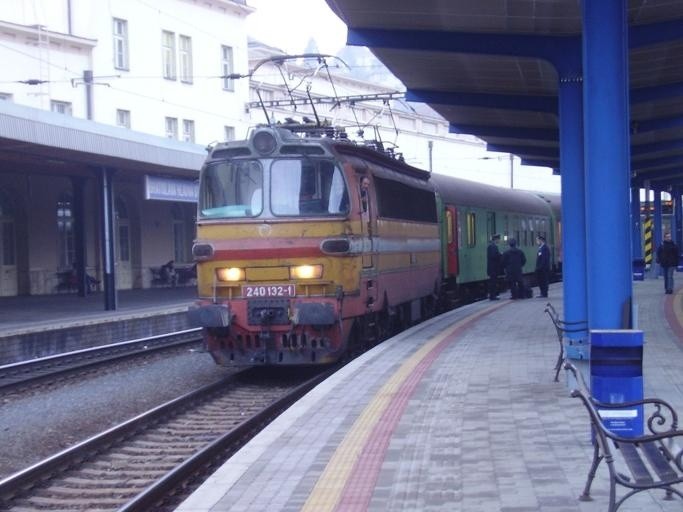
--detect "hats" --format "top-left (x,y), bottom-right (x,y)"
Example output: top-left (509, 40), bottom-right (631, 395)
top-left (492, 233), bottom-right (502, 241)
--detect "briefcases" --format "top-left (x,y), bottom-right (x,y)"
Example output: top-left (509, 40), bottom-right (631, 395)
top-left (513, 287), bottom-right (533, 298)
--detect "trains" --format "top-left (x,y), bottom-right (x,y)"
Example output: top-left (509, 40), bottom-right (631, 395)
top-left (185, 51), bottom-right (681, 378)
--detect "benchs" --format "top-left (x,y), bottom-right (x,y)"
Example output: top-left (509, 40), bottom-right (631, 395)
top-left (543, 302), bottom-right (683, 512)
top-left (53, 266), bottom-right (198, 293)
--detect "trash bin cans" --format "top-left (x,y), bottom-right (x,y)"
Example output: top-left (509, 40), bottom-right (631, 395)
top-left (676, 252), bottom-right (683, 272)
top-left (633, 257), bottom-right (645, 280)
top-left (589, 330), bottom-right (643, 448)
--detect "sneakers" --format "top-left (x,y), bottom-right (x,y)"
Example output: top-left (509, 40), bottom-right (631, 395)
top-left (490, 294), bottom-right (500, 300)
top-left (665, 289), bottom-right (674, 295)
top-left (536, 294), bottom-right (547, 298)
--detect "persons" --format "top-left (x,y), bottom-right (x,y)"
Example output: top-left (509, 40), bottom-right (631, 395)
top-left (339, 177), bottom-right (369, 211)
top-left (487, 234), bottom-right (502, 300)
top-left (535, 235), bottom-right (550, 298)
top-left (502, 239), bottom-right (526, 299)
top-left (655, 233), bottom-right (678, 293)
